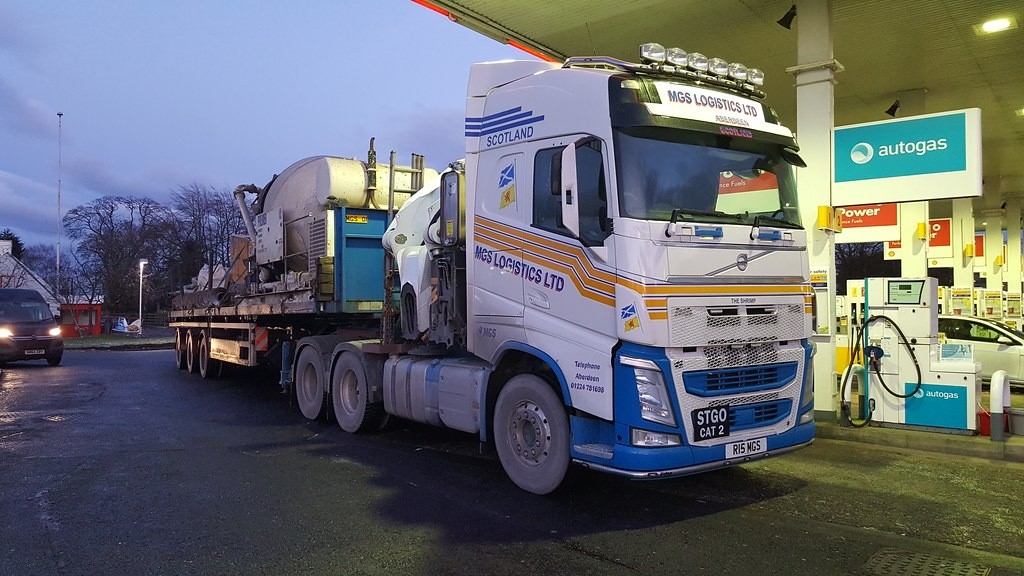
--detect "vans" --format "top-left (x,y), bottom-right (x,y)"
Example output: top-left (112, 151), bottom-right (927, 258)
top-left (0, 289), bottom-right (64, 366)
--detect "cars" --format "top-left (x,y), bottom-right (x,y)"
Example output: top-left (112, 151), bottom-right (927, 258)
top-left (938, 314), bottom-right (1024, 390)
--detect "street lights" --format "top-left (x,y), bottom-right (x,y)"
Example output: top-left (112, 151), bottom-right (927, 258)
top-left (139, 260), bottom-right (148, 334)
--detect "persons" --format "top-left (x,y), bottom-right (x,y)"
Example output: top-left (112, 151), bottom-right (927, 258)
top-left (959, 323), bottom-right (972, 336)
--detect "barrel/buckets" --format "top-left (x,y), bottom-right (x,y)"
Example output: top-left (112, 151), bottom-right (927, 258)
top-left (1004, 408), bottom-right (1024, 435)
top-left (977, 401), bottom-right (991, 436)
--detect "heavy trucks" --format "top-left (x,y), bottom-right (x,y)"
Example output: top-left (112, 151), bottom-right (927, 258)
top-left (168, 42), bottom-right (817, 497)
